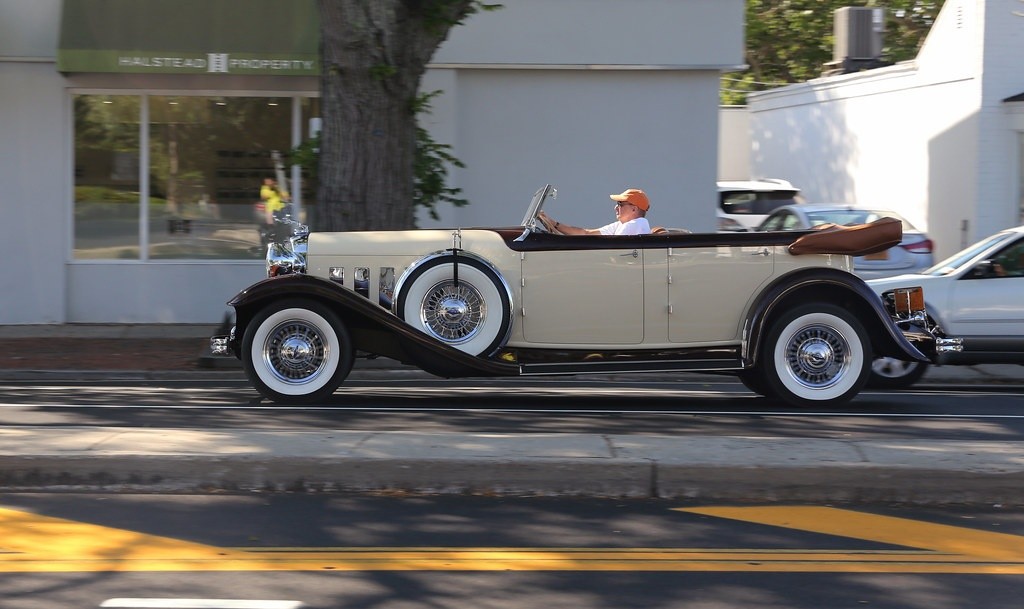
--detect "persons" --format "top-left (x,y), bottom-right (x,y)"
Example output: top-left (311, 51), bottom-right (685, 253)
top-left (541, 189), bottom-right (650, 235)
top-left (260, 176), bottom-right (290, 225)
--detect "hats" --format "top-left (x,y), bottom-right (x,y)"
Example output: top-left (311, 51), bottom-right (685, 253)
top-left (610, 188), bottom-right (650, 213)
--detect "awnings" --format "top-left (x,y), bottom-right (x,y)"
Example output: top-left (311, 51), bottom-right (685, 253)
top-left (56, 0), bottom-right (322, 78)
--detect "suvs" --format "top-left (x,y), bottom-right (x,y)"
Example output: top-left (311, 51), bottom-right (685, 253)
top-left (718, 176), bottom-right (806, 232)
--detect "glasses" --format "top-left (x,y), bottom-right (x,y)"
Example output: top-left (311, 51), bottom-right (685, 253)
top-left (616, 201), bottom-right (634, 208)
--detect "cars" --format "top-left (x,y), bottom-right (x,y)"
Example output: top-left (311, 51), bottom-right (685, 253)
top-left (759, 205), bottom-right (935, 282)
top-left (209, 180), bottom-right (961, 413)
top-left (861, 225), bottom-right (1024, 391)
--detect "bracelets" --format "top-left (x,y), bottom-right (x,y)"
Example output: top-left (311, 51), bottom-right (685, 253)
top-left (554, 222), bottom-right (559, 228)
